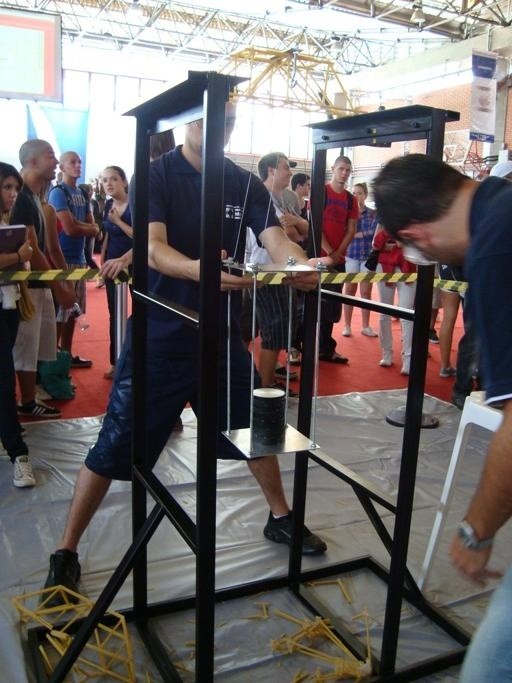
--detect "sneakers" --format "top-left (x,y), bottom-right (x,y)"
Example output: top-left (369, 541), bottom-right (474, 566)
top-left (451, 393), bottom-right (467, 409)
top-left (287, 353), bottom-right (301, 366)
top-left (380, 356), bottom-right (393, 366)
top-left (318, 350), bottom-right (349, 362)
top-left (271, 383), bottom-right (298, 399)
top-left (342, 328), bottom-right (351, 337)
top-left (95, 278), bottom-right (105, 287)
top-left (41, 549), bottom-right (80, 609)
top-left (263, 511), bottom-right (327, 556)
top-left (273, 367), bottom-right (297, 380)
top-left (402, 357), bottom-right (411, 373)
top-left (361, 328), bottom-right (378, 337)
top-left (69, 356), bottom-right (93, 367)
top-left (440, 367), bottom-right (458, 377)
top-left (429, 330), bottom-right (439, 344)
top-left (13, 456), bottom-right (36, 488)
top-left (17, 403), bottom-right (61, 416)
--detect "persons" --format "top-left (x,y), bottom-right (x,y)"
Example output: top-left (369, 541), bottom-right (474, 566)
top-left (290, 173), bottom-right (311, 223)
top-left (99, 164), bottom-right (132, 379)
top-left (47, 150), bottom-right (100, 369)
top-left (88, 183), bottom-right (104, 240)
top-left (78, 183), bottom-right (105, 289)
top-left (428, 267), bottom-right (442, 345)
top-left (341, 183), bottom-right (379, 339)
top-left (371, 222), bottom-right (418, 377)
top-left (8, 138), bottom-right (77, 418)
top-left (306, 155), bottom-right (359, 364)
top-left (0, 161), bottom-right (37, 488)
top-left (39, 97), bottom-right (329, 609)
top-left (451, 296), bottom-right (484, 411)
top-left (19, 167), bottom-right (77, 400)
top-left (372, 152), bottom-right (512, 683)
top-left (244, 152), bottom-right (310, 397)
top-left (438, 265), bottom-right (463, 378)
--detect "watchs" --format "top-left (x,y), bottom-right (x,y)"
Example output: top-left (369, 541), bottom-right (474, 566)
top-left (455, 517), bottom-right (495, 554)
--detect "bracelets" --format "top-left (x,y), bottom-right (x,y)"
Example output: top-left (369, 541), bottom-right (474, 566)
top-left (16, 251), bottom-right (21, 266)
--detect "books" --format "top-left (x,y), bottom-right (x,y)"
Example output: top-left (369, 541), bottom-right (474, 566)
top-left (0, 223), bottom-right (27, 271)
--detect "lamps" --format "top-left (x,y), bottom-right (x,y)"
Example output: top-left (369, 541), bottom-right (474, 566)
top-left (409, 5), bottom-right (426, 28)
